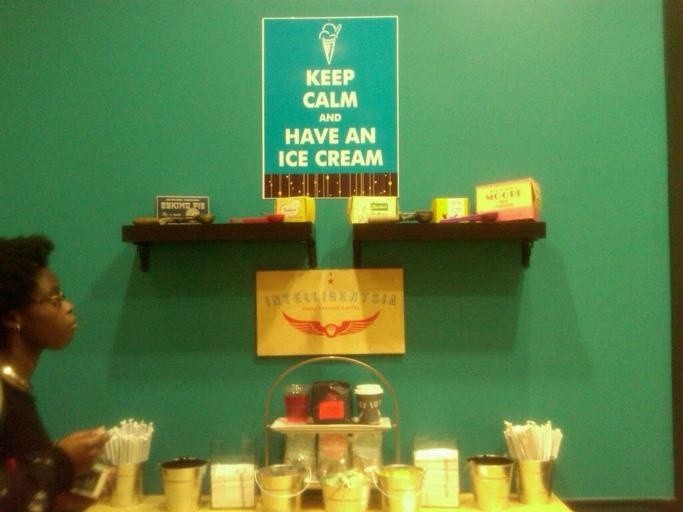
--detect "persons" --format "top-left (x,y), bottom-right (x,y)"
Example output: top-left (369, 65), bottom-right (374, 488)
top-left (0, 233), bottom-right (110, 511)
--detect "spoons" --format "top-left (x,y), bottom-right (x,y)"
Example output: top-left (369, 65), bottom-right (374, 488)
top-left (370, 208), bottom-right (434, 223)
top-left (229, 214), bottom-right (285, 226)
top-left (132, 212), bottom-right (214, 226)
top-left (438, 209), bottom-right (499, 224)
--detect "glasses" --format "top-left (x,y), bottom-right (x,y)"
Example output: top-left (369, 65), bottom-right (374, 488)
top-left (31, 290), bottom-right (63, 306)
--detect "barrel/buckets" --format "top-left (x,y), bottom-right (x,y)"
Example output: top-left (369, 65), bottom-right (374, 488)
top-left (516, 459), bottom-right (557, 505)
top-left (320, 474), bottom-right (372, 511)
top-left (374, 464), bottom-right (427, 511)
top-left (254, 464), bottom-right (311, 512)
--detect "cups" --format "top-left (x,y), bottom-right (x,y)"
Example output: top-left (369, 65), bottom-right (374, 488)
top-left (515, 460), bottom-right (551, 505)
top-left (353, 383), bottom-right (384, 425)
top-left (281, 382), bottom-right (310, 424)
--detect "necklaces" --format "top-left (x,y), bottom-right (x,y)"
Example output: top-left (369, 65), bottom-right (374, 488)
top-left (1, 363), bottom-right (30, 390)
